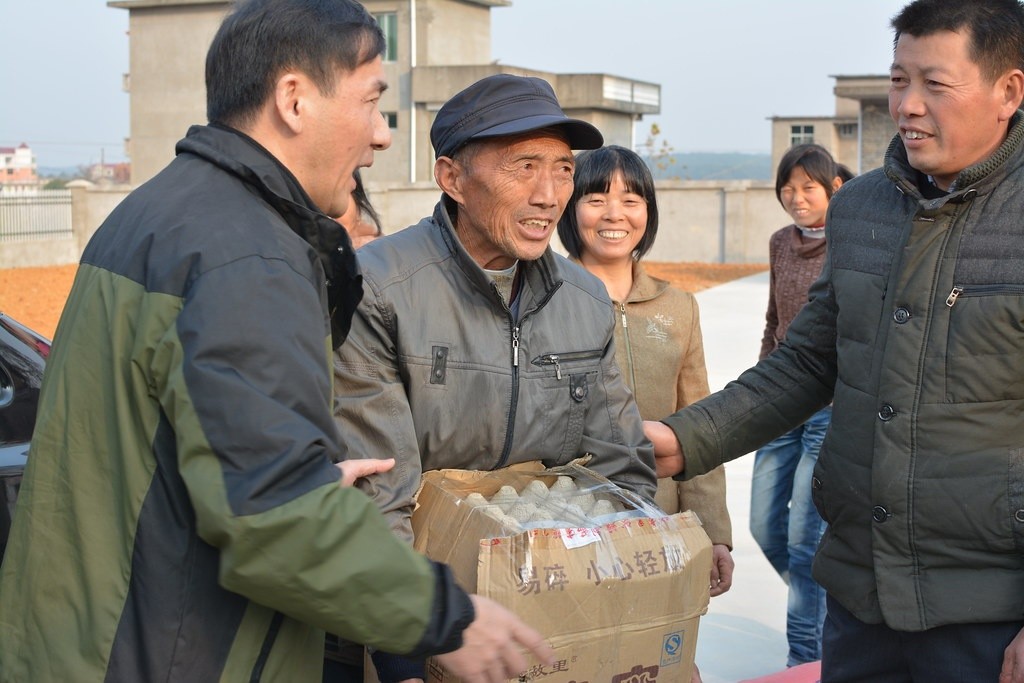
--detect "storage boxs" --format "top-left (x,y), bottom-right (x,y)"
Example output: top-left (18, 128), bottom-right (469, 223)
top-left (363, 453), bottom-right (713, 683)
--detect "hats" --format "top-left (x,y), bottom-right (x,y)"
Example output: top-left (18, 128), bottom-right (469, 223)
top-left (430, 74), bottom-right (604, 163)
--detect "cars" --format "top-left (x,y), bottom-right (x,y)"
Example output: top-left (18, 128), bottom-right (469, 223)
top-left (0, 308), bottom-right (53, 573)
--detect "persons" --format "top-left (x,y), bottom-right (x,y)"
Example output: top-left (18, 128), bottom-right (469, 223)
top-left (0, 0), bottom-right (734, 683)
top-left (642, 0), bottom-right (1024, 683)
